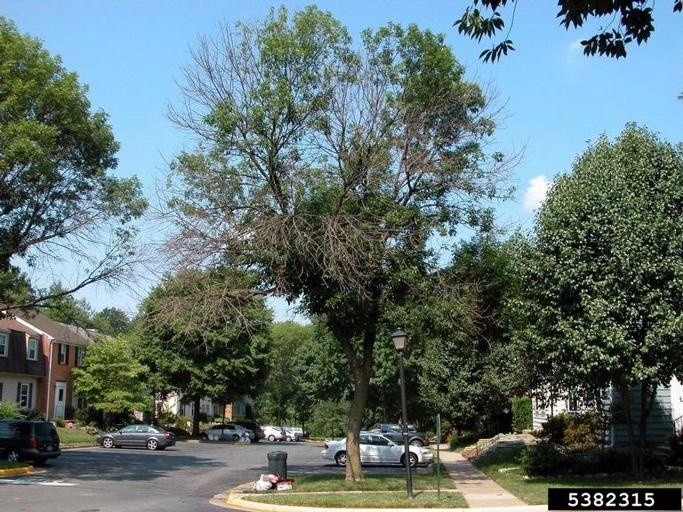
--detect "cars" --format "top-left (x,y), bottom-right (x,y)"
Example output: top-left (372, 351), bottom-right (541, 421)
top-left (259, 425), bottom-right (306, 442)
top-left (96, 424), bottom-right (176, 450)
top-left (199, 424), bottom-right (255, 441)
top-left (320, 424), bottom-right (434, 467)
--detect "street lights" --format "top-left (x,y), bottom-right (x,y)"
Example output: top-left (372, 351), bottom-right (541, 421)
top-left (391, 327), bottom-right (415, 498)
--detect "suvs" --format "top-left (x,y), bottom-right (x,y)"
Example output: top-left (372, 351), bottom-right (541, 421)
top-left (0, 420), bottom-right (62, 465)
top-left (227, 421), bottom-right (266, 442)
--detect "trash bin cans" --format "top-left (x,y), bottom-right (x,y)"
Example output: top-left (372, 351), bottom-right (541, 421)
top-left (267, 451), bottom-right (287, 482)
